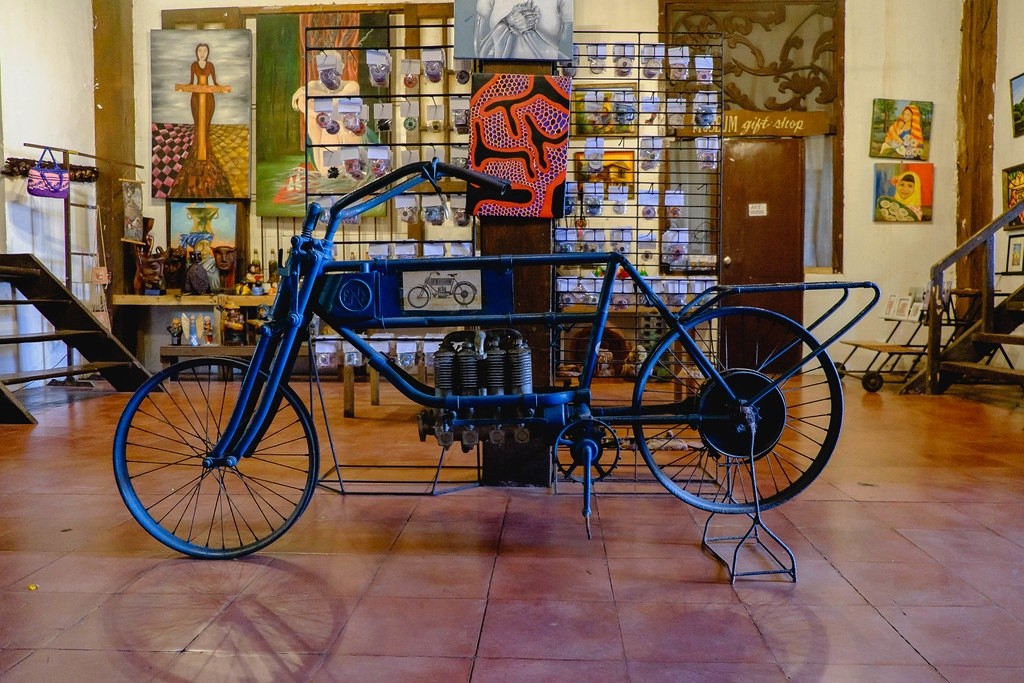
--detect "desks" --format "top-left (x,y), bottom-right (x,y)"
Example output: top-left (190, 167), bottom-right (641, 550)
top-left (562, 304), bottom-right (697, 401)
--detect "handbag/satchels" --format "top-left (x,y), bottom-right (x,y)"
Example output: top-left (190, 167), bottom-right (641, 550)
top-left (27, 147), bottom-right (69, 199)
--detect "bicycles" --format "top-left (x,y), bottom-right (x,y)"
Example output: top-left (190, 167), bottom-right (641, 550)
top-left (110, 159), bottom-right (880, 596)
top-left (408, 273), bottom-right (477, 308)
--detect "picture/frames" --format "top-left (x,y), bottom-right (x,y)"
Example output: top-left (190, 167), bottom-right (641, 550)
top-left (1002, 163), bottom-right (1024, 231)
top-left (574, 151), bottom-right (635, 200)
top-left (1006, 234), bottom-right (1024, 272)
top-left (894, 296), bottom-right (914, 320)
top-left (882, 294), bottom-right (899, 317)
top-left (908, 302), bottom-right (923, 320)
top-left (571, 82), bottom-right (639, 137)
top-left (1008, 72), bottom-right (1024, 138)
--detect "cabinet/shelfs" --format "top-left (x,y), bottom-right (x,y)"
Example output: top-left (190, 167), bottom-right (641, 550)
top-left (112, 294), bottom-right (315, 381)
top-left (831, 292), bottom-right (1024, 393)
